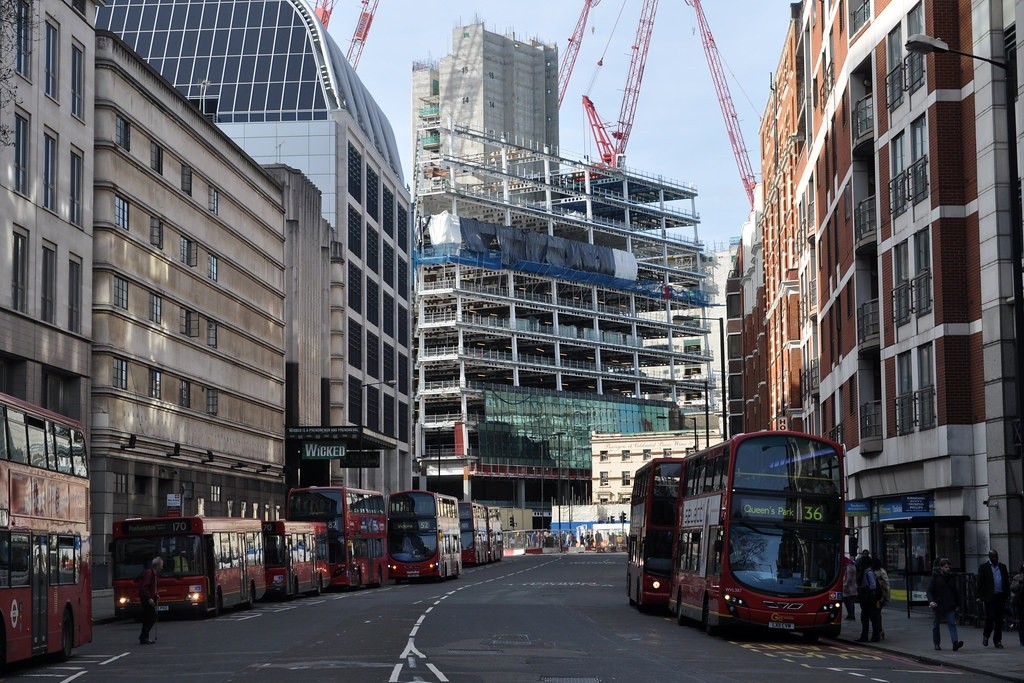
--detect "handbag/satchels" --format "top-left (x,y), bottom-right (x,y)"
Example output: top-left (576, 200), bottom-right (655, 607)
top-left (857, 570), bottom-right (884, 606)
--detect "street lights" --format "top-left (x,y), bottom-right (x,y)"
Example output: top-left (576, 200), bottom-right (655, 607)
top-left (904, 34), bottom-right (1024, 450)
top-left (437, 426), bottom-right (443, 495)
top-left (671, 315), bottom-right (727, 442)
top-left (359, 379), bottom-right (397, 489)
top-left (661, 379), bottom-right (710, 449)
top-left (551, 431), bottom-right (567, 530)
top-left (655, 416), bottom-right (697, 453)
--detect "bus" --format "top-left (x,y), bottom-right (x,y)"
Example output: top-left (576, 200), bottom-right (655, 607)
top-left (285, 485), bottom-right (388, 592)
top-left (388, 490), bottom-right (462, 585)
top-left (261, 520), bottom-right (331, 602)
top-left (107, 515), bottom-right (266, 622)
top-left (627, 456), bottom-right (685, 616)
top-left (668, 430), bottom-right (860, 643)
top-left (0, 392), bottom-right (92, 679)
top-left (484, 506), bottom-right (503, 564)
top-left (457, 502), bottom-right (489, 567)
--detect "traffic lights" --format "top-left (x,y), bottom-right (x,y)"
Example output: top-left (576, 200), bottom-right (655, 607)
top-left (611, 515), bottom-right (615, 523)
top-left (622, 511), bottom-right (627, 523)
top-left (509, 516), bottom-right (515, 526)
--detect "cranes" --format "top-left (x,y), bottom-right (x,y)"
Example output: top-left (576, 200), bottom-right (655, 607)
top-left (685, 0), bottom-right (757, 211)
top-left (582, 0), bottom-right (659, 170)
top-left (346, 0), bottom-right (380, 71)
top-left (557, 1), bottom-right (602, 111)
top-left (314, 0), bottom-right (334, 31)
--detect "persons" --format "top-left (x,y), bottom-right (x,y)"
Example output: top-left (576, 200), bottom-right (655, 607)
top-left (139, 557), bottom-right (164, 643)
top-left (799, 470), bottom-right (835, 495)
top-left (169, 549), bottom-right (188, 573)
top-left (927, 560), bottom-right (964, 651)
top-left (843, 550), bottom-right (890, 642)
top-left (976, 549), bottom-right (1011, 649)
top-left (508, 531), bottom-right (614, 547)
top-left (1010, 567), bottom-right (1024, 646)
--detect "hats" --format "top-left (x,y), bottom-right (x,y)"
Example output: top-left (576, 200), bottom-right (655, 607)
top-left (855, 556), bottom-right (872, 570)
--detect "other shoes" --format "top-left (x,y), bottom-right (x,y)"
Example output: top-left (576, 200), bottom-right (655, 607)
top-left (953, 641), bottom-right (963, 651)
top-left (845, 616), bottom-right (856, 621)
top-left (140, 639), bottom-right (155, 645)
top-left (860, 634), bottom-right (868, 641)
top-left (871, 631), bottom-right (881, 642)
top-left (995, 640), bottom-right (1003, 649)
top-left (935, 644), bottom-right (941, 650)
top-left (983, 635), bottom-right (988, 646)
